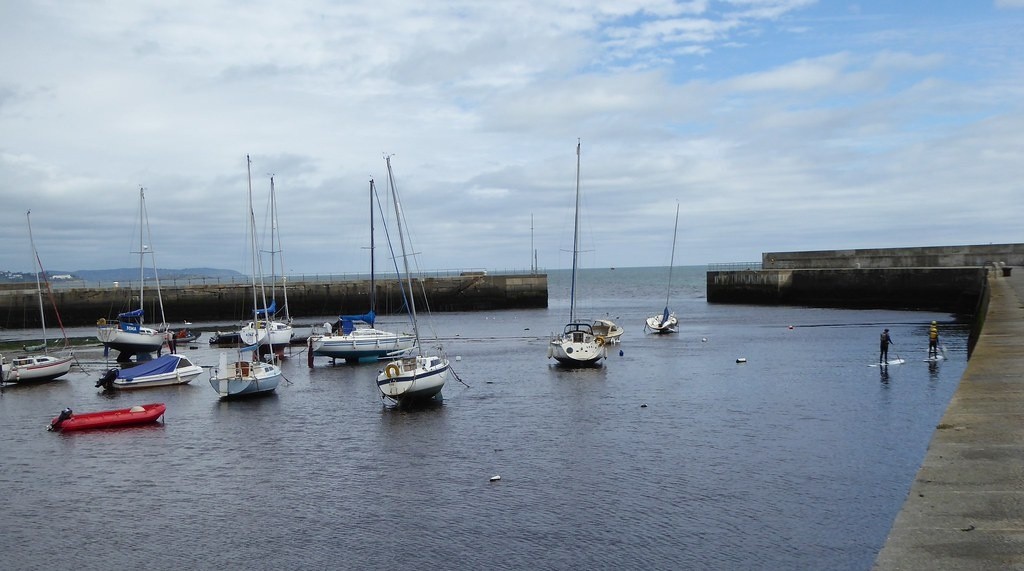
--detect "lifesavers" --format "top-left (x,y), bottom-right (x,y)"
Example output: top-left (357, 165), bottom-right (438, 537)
top-left (385, 364), bottom-right (400, 378)
top-left (594, 335), bottom-right (605, 348)
top-left (96, 318), bottom-right (106, 327)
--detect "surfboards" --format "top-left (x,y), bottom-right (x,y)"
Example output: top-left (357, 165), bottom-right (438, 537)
top-left (868, 359), bottom-right (905, 367)
top-left (923, 354), bottom-right (944, 363)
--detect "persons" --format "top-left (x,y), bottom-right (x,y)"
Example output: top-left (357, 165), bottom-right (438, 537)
top-left (927, 320), bottom-right (940, 359)
top-left (323, 321), bottom-right (333, 337)
top-left (879, 328), bottom-right (894, 365)
top-left (167, 331), bottom-right (177, 354)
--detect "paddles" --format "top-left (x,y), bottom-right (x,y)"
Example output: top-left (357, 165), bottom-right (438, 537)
top-left (893, 344), bottom-right (901, 362)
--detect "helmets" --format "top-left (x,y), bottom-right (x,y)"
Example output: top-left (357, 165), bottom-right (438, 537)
top-left (932, 321), bottom-right (936, 325)
top-left (884, 329), bottom-right (889, 332)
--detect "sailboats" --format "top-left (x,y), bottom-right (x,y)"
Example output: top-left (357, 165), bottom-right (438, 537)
top-left (549, 138), bottom-right (605, 365)
top-left (239, 173), bottom-right (292, 355)
top-left (646, 202), bottom-right (680, 333)
top-left (377, 152), bottom-right (449, 405)
top-left (96, 187), bottom-right (170, 355)
top-left (306, 175), bottom-right (417, 358)
top-left (0, 207), bottom-right (75, 382)
top-left (207, 153), bottom-right (282, 398)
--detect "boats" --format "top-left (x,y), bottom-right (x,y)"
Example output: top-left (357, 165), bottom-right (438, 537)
top-left (163, 329), bottom-right (202, 344)
top-left (585, 311), bottom-right (625, 344)
top-left (210, 330), bottom-right (243, 344)
top-left (95, 352), bottom-right (204, 393)
top-left (48, 400), bottom-right (168, 435)
